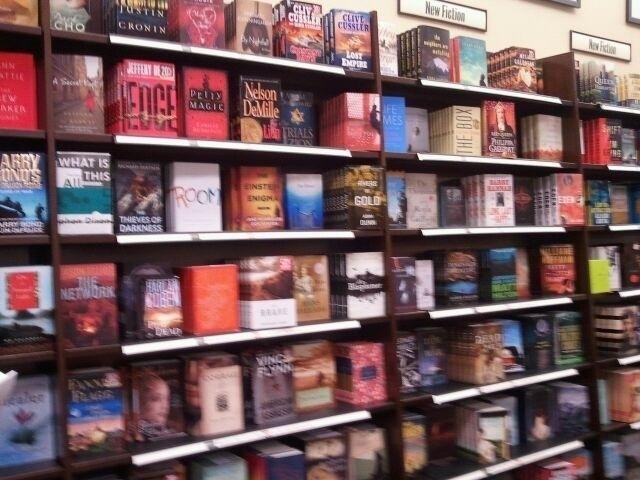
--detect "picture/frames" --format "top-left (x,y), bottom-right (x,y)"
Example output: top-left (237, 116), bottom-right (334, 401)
top-left (570, 30), bottom-right (631, 62)
top-left (550, 0), bottom-right (582, 8)
top-left (396, 0), bottom-right (487, 31)
top-left (623, 0), bottom-right (639, 24)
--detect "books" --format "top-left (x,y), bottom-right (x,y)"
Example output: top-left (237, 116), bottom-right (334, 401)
top-left (50, 0), bottom-right (390, 479)
top-left (377, 25), bottom-right (639, 480)
top-left (1, 0), bottom-right (56, 468)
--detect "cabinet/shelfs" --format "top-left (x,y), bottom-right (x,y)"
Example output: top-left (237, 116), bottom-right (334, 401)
top-left (1, 0), bottom-right (640, 479)
